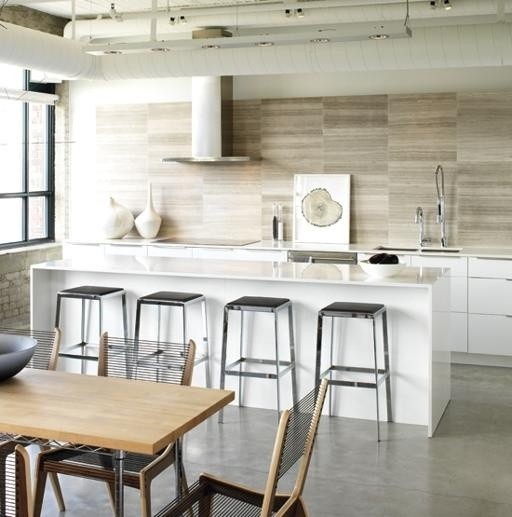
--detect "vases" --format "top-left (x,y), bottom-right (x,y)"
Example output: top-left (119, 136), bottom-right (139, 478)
top-left (134, 180), bottom-right (163, 237)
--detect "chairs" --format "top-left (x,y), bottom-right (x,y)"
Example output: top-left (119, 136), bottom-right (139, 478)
top-left (151, 374), bottom-right (328, 517)
top-left (0, 324), bottom-right (197, 517)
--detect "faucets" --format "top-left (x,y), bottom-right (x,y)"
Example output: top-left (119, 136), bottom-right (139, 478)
top-left (414, 205), bottom-right (428, 248)
top-left (434, 164), bottom-right (447, 248)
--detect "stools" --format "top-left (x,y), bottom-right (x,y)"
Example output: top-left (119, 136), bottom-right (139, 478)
top-left (218, 295), bottom-right (298, 425)
top-left (314, 301), bottom-right (392, 443)
top-left (54, 286), bottom-right (131, 371)
top-left (134, 291), bottom-right (211, 387)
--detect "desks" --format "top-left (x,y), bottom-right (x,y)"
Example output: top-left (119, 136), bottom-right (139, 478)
top-left (0, 366), bottom-right (234, 517)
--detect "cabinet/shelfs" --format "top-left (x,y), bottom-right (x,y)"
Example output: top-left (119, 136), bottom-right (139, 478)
top-left (411, 254), bottom-right (469, 357)
top-left (470, 257), bottom-right (512, 356)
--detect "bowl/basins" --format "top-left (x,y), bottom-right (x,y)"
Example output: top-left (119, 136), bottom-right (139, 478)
top-left (360, 259), bottom-right (407, 278)
top-left (1, 329), bottom-right (37, 378)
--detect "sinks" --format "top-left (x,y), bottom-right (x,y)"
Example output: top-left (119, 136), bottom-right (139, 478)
top-left (374, 244), bottom-right (462, 255)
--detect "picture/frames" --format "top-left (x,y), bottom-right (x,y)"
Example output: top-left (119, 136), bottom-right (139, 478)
top-left (292, 169), bottom-right (354, 247)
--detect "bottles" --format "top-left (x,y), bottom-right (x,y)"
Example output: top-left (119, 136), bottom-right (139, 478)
top-left (272, 203), bottom-right (283, 240)
